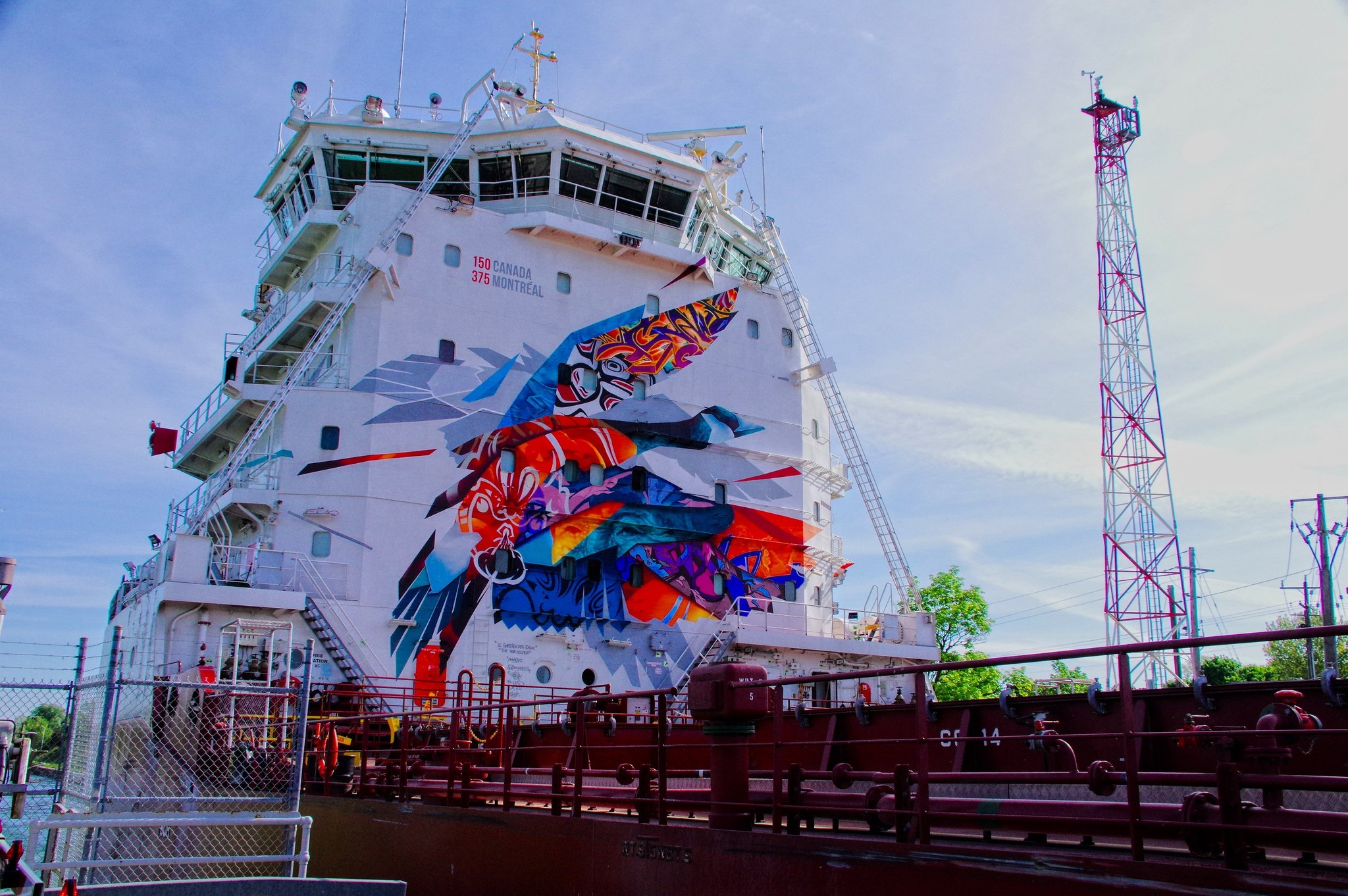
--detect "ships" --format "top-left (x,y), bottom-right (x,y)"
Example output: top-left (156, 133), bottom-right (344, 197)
top-left (1, 17), bottom-right (1346, 895)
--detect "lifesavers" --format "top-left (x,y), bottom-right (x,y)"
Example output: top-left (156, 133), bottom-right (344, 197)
top-left (315, 720), bottom-right (339, 780)
